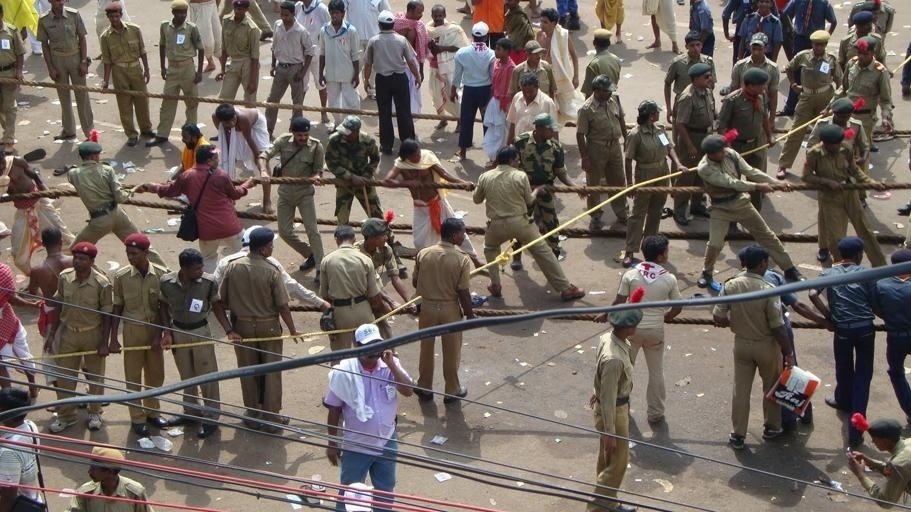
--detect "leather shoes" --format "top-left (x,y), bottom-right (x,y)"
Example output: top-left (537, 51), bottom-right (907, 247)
top-left (300, 256), bottom-right (314, 269)
top-left (609, 504), bottom-right (634, 512)
top-left (590, 208), bottom-right (711, 230)
top-left (896, 204), bottom-right (911, 215)
top-left (414, 385), bottom-right (433, 401)
top-left (698, 274), bottom-right (712, 287)
top-left (4, 130), bottom-right (218, 154)
top-left (47, 394), bottom-right (290, 438)
top-left (397, 263), bottom-right (407, 272)
top-left (817, 250), bottom-right (829, 261)
top-left (314, 271), bottom-right (320, 280)
top-left (444, 385), bottom-right (467, 403)
top-left (487, 261), bottom-right (585, 299)
top-left (622, 252), bottom-right (633, 266)
top-left (729, 394), bottom-right (864, 450)
top-left (784, 267), bottom-right (806, 282)
top-left (776, 167), bottom-right (786, 179)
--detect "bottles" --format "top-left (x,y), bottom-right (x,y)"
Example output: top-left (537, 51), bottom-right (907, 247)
top-left (708, 279), bottom-right (725, 293)
top-left (472, 291), bottom-right (490, 307)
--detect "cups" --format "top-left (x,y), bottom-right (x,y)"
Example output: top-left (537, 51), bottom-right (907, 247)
top-left (310, 475), bottom-right (323, 490)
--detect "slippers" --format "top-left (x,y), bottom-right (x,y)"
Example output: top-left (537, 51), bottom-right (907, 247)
top-left (299, 486), bottom-right (319, 505)
top-left (471, 295), bottom-right (487, 307)
top-left (311, 474), bottom-right (326, 491)
top-left (53, 165), bottom-right (72, 175)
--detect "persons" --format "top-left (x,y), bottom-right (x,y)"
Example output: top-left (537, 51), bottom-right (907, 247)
top-left (63, 445), bottom-right (153, 510)
top-left (846, 418), bottom-right (909, 511)
top-left (327, 324), bottom-right (414, 512)
top-left (584, 302), bottom-right (641, 511)
top-left (0, 1), bottom-right (911, 456)
top-left (0, 385), bottom-right (44, 510)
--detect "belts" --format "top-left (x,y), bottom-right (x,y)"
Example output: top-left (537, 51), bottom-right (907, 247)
top-left (596, 397), bottom-right (629, 405)
top-left (91, 202), bottom-right (115, 217)
top-left (334, 295), bottom-right (365, 306)
top-left (64, 317), bottom-right (278, 332)
top-left (887, 331), bottom-right (911, 336)
top-left (838, 320), bottom-right (872, 328)
top-left (0, 48), bottom-right (294, 71)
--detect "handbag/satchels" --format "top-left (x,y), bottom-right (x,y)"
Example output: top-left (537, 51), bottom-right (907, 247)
top-left (176, 206), bottom-right (198, 241)
top-left (12, 496), bottom-right (45, 512)
top-left (273, 163), bottom-right (282, 177)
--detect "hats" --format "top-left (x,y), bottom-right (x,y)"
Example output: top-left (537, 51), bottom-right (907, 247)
top-left (838, 237), bottom-right (864, 255)
top-left (354, 323), bottom-right (384, 344)
top-left (125, 234), bottom-right (150, 249)
top-left (687, 1), bottom-right (881, 153)
top-left (890, 250), bottom-right (911, 263)
top-left (608, 302), bottom-right (642, 327)
top-left (378, 10), bottom-right (394, 23)
top-left (525, 29), bottom-right (662, 127)
top-left (868, 418), bottom-right (903, 436)
top-left (241, 217), bottom-right (387, 245)
top-left (72, 241), bottom-right (97, 258)
top-left (472, 22), bottom-right (489, 37)
top-left (292, 117), bottom-right (310, 132)
top-left (105, 1), bottom-right (250, 10)
top-left (92, 446), bottom-right (124, 467)
top-left (336, 114), bottom-right (361, 135)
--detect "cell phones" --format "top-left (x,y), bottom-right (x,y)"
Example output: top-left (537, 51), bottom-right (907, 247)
top-left (381, 352), bottom-right (387, 359)
top-left (847, 446), bottom-right (854, 455)
